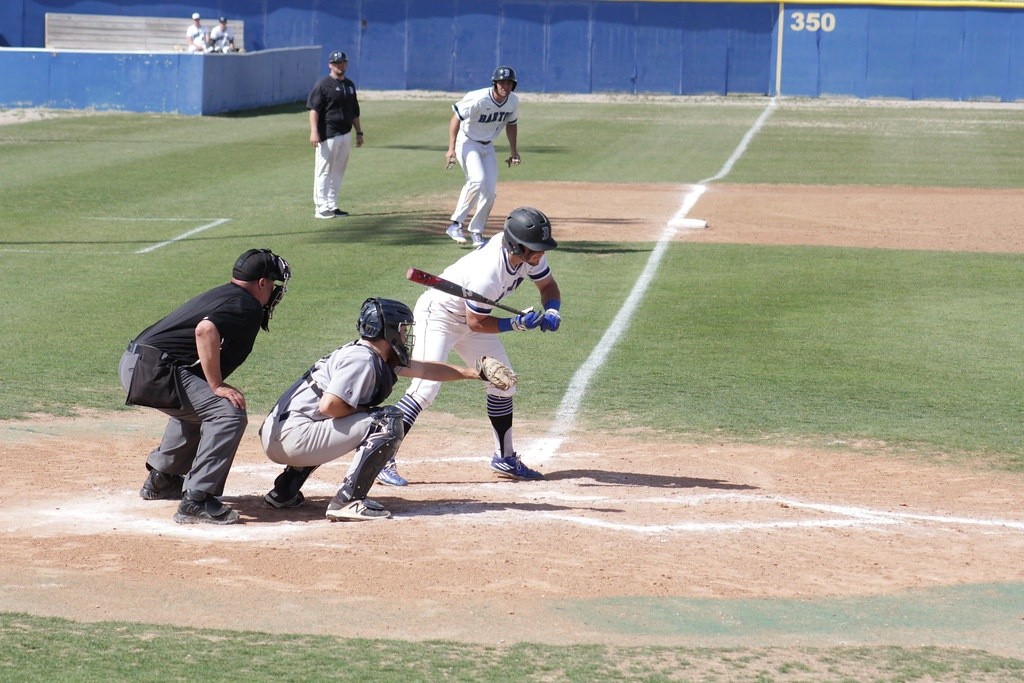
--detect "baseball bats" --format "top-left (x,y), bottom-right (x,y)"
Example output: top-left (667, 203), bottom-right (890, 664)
top-left (405, 265), bottom-right (542, 325)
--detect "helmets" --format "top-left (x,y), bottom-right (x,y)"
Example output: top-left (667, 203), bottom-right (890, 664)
top-left (491, 67), bottom-right (518, 91)
top-left (355, 298), bottom-right (416, 369)
top-left (504, 207), bottom-right (558, 256)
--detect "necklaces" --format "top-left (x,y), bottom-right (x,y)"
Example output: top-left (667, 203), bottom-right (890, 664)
top-left (508, 253), bottom-right (526, 278)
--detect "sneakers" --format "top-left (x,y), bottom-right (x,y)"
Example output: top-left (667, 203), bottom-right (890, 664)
top-left (263, 489), bottom-right (305, 508)
top-left (470, 230), bottom-right (484, 246)
top-left (315, 209), bottom-right (336, 219)
top-left (375, 460), bottom-right (407, 486)
top-left (332, 208), bottom-right (348, 215)
top-left (326, 494), bottom-right (391, 521)
top-left (490, 452), bottom-right (544, 480)
top-left (173, 496), bottom-right (240, 526)
top-left (139, 468), bottom-right (185, 500)
top-left (446, 224), bottom-right (467, 247)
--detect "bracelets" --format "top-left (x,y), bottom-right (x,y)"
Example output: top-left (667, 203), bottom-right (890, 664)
top-left (357, 132), bottom-right (363, 136)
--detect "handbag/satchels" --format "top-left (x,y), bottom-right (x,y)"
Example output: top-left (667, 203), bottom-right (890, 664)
top-left (125, 345), bottom-right (184, 411)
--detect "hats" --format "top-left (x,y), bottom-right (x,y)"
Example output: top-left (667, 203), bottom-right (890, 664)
top-left (192, 12), bottom-right (200, 20)
top-left (232, 248), bottom-right (280, 281)
top-left (219, 17), bottom-right (226, 23)
top-left (327, 50), bottom-right (349, 63)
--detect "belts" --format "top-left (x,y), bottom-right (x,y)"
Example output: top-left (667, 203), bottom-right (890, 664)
top-left (279, 411), bottom-right (290, 422)
top-left (127, 344), bottom-right (141, 355)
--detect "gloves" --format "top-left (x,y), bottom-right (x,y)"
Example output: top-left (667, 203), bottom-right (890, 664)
top-left (541, 308), bottom-right (560, 332)
top-left (509, 306), bottom-right (545, 332)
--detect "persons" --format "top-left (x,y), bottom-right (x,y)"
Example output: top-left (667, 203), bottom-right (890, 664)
top-left (306, 50), bottom-right (364, 218)
top-left (446, 67), bottom-right (521, 246)
top-left (209, 16), bottom-right (234, 53)
top-left (259, 297), bottom-right (518, 520)
top-left (119, 249), bottom-right (292, 524)
top-left (186, 13), bottom-right (209, 53)
top-left (375, 207), bottom-right (562, 486)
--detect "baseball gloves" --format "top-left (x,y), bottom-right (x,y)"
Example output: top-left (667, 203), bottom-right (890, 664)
top-left (476, 354), bottom-right (518, 391)
